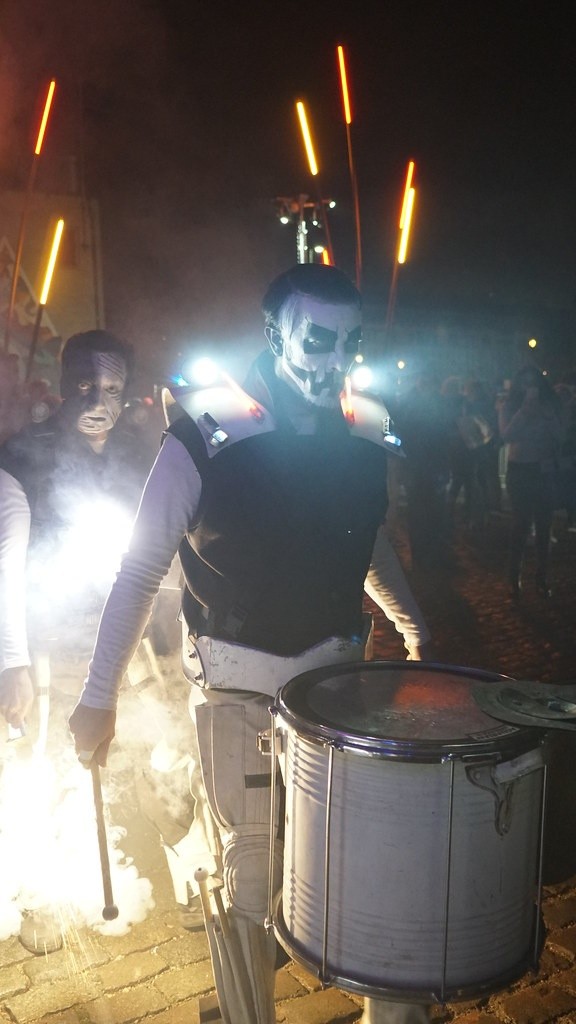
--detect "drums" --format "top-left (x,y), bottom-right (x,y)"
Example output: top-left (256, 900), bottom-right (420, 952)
top-left (252, 656), bottom-right (550, 1005)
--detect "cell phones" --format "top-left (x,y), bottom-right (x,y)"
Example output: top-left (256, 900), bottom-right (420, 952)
top-left (526, 387), bottom-right (539, 400)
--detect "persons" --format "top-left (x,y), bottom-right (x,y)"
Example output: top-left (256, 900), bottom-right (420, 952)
top-left (65, 268), bottom-right (448, 1024)
top-left (0, 328), bottom-right (193, 951)
top-left (339, 364), bottom-right (576, 599)
